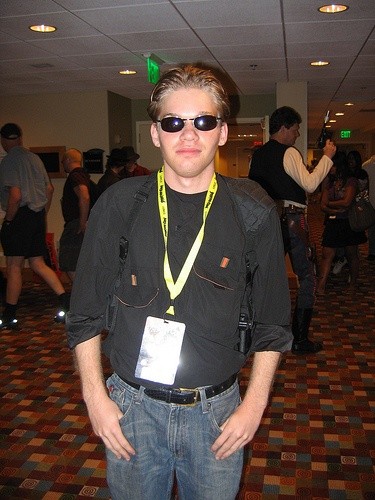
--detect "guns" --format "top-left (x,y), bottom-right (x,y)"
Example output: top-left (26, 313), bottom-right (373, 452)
top-left (319, 110), bottom-right (333, 146)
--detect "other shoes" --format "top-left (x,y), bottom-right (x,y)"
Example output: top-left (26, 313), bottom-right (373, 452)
top-left (332, 255), bottom-right (349, 274)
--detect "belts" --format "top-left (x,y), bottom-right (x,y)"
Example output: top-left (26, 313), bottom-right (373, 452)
top-left (115, 370), bottom-right (238, 407)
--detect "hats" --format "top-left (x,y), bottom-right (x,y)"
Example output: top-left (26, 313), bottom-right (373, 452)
top-left (0, 122), bottom-right (23, 141)
top-left (107, 145), bottom-right (140, 169)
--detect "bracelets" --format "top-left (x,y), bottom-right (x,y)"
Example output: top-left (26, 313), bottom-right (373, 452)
top-left (4, 217), bottom-right (12, 223)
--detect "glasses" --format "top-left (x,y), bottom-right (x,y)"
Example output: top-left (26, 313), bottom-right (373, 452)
top-left (156, 114), bottom-right (221, 134)
top-left (60, 157), bottom-right (68, 164)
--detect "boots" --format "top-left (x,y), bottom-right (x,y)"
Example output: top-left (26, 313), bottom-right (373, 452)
top-left (290, 305), bottom-right (325, 356)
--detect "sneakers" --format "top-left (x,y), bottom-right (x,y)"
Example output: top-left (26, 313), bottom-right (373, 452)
top-left (0, 313), bottom-right (19, 330)
top-left (53, 307), bottom-right (68, 323)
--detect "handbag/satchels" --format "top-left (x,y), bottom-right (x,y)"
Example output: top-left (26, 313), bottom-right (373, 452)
top-left (347, 198), bottom-right (375, 233)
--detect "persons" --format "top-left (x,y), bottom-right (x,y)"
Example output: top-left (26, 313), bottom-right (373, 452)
top-left (315, 150), bottom-right (375, 296)
top-left (64, 63), bottom-right (295, 500)
top-left (248, 106), bottom-right (337, 354)
top-left (97, 145), bottom-right (152, 198)
top-left (58, 148), bottom-right (98, 285)
top-left (0, 122), bottom-right (71, 330)
